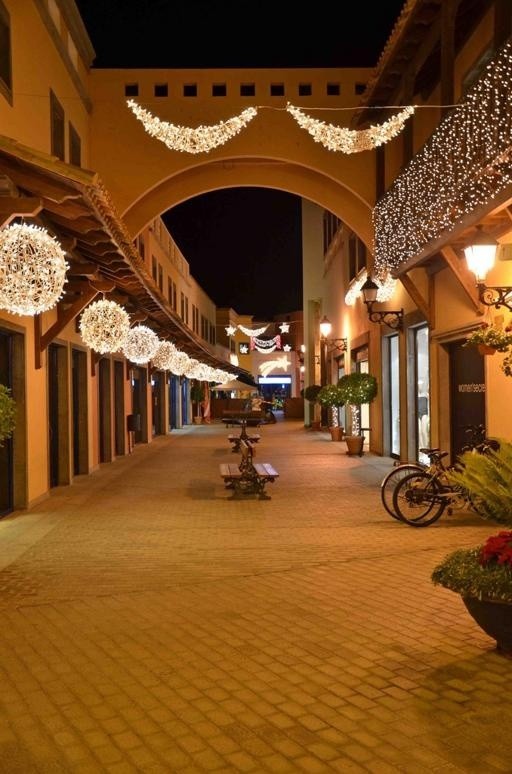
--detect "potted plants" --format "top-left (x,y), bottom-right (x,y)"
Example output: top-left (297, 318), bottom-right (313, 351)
top-left (462, 325), bottom-right (511, 378)
top-left (190, 383), bottom-right (205, 424)
top-left (298, 372), bottom-right (378, 457)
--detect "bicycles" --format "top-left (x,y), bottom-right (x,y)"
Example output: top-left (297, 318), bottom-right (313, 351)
top-left (392, 423), bottom-right (501, 528)
top-left (381, 424), bottom-right (490, 521)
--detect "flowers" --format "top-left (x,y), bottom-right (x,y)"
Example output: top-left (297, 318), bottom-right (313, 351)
top-left (431, 526), bottom-right (512, 598)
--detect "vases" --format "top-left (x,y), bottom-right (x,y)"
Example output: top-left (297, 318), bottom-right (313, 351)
top-left (459, 599), bottom-right (512, 659)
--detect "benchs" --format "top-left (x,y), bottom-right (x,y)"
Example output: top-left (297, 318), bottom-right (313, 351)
top-left (218, 421), bottom-right (279, 502)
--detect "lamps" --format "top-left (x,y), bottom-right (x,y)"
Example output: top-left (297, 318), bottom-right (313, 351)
top-left (459, 222), bottom-right (512, 312)
top-left (319, 314), bottom-right (348, 351)
top-left (1, 217), bottom-right (70, 317)
top-left (358, 275), bottom-right (405, 334)
top-left (76, 298), bottom-right (241, 385)
